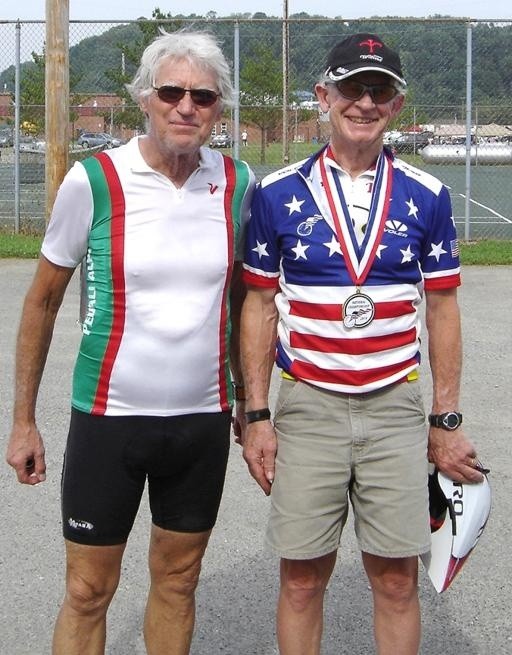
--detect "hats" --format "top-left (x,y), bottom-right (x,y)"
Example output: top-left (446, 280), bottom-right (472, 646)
top-left (324, 33), bottom-right (408, 94)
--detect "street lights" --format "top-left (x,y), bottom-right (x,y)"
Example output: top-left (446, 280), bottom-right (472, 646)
top-left (289, 101), bottom-right (302, 143)
top-left (93, 100), bottom-right (113, 135)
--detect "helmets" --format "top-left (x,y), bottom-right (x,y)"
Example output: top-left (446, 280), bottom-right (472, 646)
top-left (409, 446), bottom-right (491, 594)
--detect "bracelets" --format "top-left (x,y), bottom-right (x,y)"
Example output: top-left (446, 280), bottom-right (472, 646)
top-left (232, 380), bottom-right (244, 401)
top-left (244, 408), bottom-right (271, 423)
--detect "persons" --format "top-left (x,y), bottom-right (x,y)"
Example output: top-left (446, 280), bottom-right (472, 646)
top-left (238, 33), bottom-right (484, 655)
top-left (241, 129), bottom-right (248, 146)
top-left (6, 33), bottom-right (256, 655)
top-left (104, 141), bottom-right (112, 149)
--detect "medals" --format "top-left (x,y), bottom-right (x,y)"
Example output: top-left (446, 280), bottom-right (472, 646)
top-left (342, 292), bottom-right (375, 330)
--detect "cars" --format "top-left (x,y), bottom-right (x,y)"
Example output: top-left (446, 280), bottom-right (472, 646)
top-left (208, 134), bottom-right (233, 149)
top-left (383, 130), bottom-right (512, 154)
top-left (0, 127), bottom-right (46, 154)
top-left (77, 132), bottom-right (120, 150)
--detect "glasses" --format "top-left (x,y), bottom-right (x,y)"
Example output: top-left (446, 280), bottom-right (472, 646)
top-left (335, 78), bottom-right (399, 104)
top-left (151, 86), bottom-right (220, 106)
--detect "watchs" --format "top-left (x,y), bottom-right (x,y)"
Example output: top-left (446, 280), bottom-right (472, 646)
top-left (428, 411), bottom-right (463, 431)
